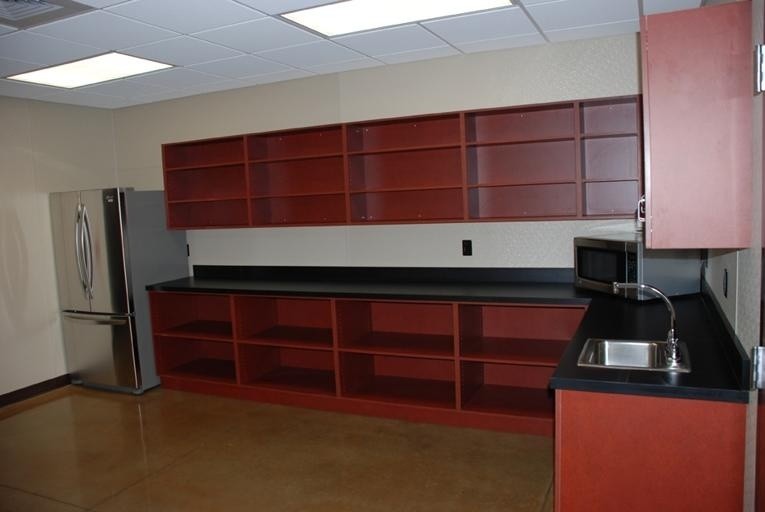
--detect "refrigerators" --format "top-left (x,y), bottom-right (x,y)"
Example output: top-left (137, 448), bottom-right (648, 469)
top-left (49, 186), bottom-right (189, 395)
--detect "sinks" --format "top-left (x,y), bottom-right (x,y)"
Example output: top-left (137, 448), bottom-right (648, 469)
top-left (578, 335), bottom-right (690, 376)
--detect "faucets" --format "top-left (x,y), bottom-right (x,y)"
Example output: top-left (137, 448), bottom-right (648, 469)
top-left (611, 279), bottom-right (680, 362)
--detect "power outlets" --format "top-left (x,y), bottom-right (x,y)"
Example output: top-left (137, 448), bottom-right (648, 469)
top-left (462, 239), bottom-right (473, 255)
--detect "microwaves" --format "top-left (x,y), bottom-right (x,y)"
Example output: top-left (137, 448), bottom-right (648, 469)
top-left (573, 232), bottom-right (701, 301)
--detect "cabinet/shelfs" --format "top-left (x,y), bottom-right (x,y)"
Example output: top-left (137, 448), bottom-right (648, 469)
top-left (642, 1), bottom-right (755, 256)
